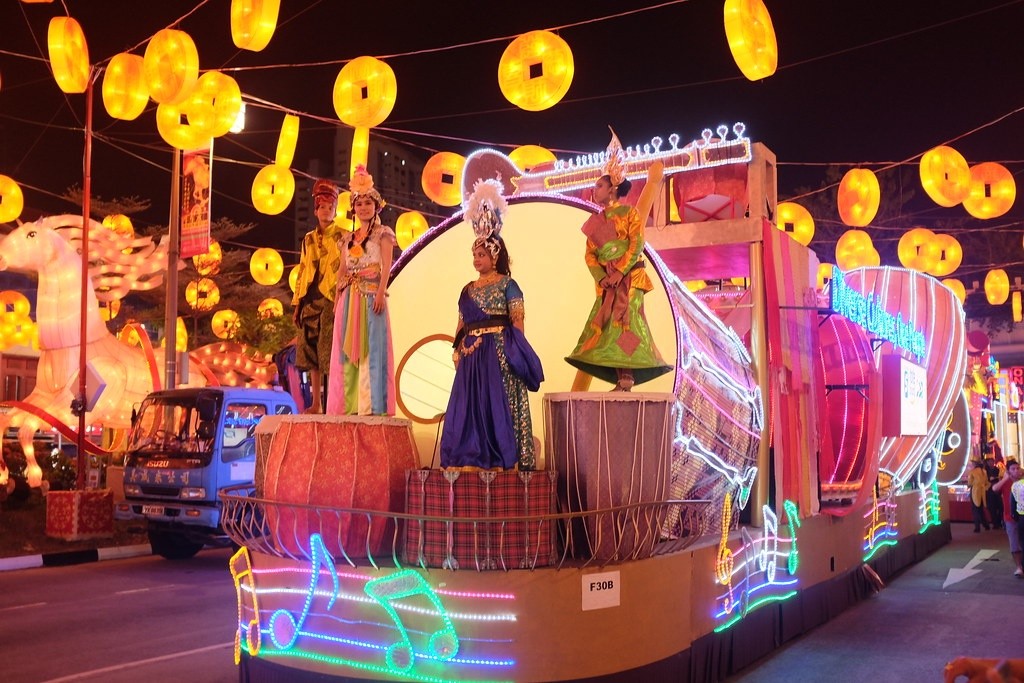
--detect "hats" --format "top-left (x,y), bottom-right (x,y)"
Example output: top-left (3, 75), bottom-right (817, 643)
top-left (970, 455), bottom-right (982, 464)
top-left (985, 454), bottom-right (993, 459)
top-left (312, 180), bottom-right (339, 208)
top-left (1007, 455), bottom-right (1015, 461)
top-left (349, 164), bottom-right (386, 213)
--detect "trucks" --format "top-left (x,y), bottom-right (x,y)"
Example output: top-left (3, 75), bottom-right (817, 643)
top-left (113, 386), bottom-right (300, 561)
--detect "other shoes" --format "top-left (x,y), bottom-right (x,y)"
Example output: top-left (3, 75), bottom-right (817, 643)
top-left (992, 525), bottom-right (1003, 529)
top-left (985, 525), bottom-right (991, 530)
top-left (1014, 567), bottom-right (1023, 576)
top-left (974, 527), bottom-right (980, 533)
top-left (608, 374), bottom-right (635, 392)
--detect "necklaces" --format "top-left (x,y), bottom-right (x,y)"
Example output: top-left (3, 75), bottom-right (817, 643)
top-left (475, 271), bottom-right (498, 286)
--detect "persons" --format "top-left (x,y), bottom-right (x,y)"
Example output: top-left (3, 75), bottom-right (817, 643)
top-left (562, 175), bottom-right (674, 392)
top-left (966, 457), bottom-right (1024, 578)
top-left (45, 443), bottom-right (75, 491)
top-left (438, 232), bottom-right (546, 471)
top-left (326, 187), bottom-right (397, 417)
top-left (290, 179), bottom-right (349, 413)
